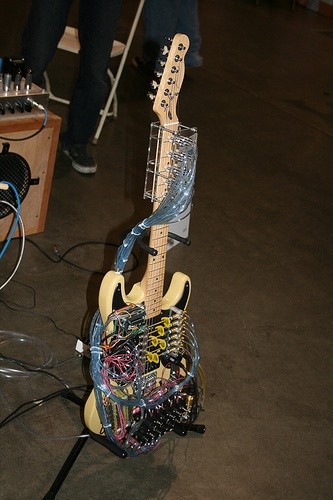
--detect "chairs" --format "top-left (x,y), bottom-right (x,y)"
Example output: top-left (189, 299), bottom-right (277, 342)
top-left (43, 0), bottom-right (146, 145)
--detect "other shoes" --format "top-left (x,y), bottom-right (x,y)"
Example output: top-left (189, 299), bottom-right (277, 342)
top-left (131, 56), bottom-right (154, 76)
top-left (61, 142), bottom-right (98, 174)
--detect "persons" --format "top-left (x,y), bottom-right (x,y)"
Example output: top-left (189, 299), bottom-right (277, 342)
top-left (17, 0), bottom-right (124, 174)
top-left (131, 0), bottom-right (205, 73)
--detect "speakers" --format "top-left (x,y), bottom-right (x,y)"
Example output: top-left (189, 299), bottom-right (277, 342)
top-left (0, 106), bottom-right (62, 242)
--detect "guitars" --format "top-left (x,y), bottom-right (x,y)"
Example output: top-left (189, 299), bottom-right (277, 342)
top-left (84, 34), bottom-right (206, 437)
